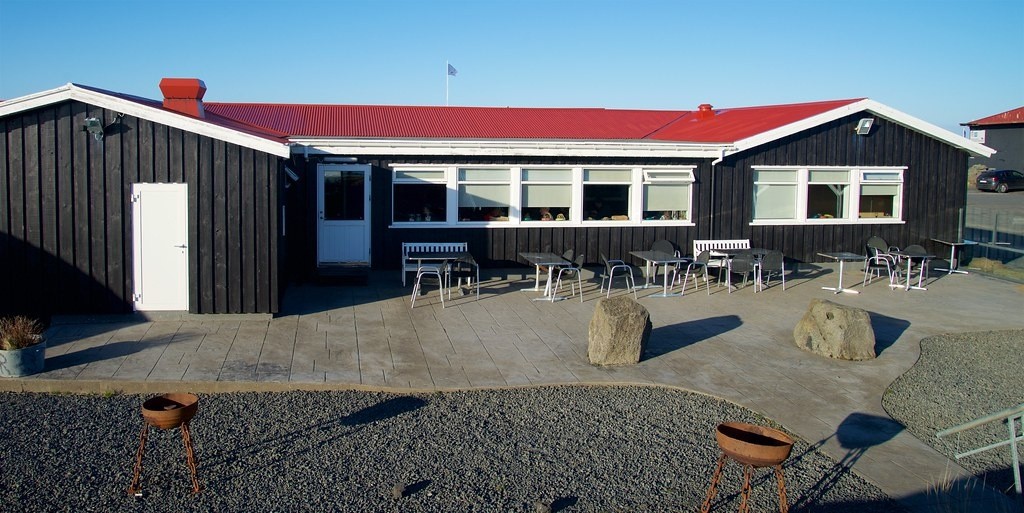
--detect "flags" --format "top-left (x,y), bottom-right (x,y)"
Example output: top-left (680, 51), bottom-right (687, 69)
top-left (448, 64), bottom-right (457, 75)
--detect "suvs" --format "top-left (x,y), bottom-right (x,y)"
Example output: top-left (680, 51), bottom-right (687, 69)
top-left (975, 170), bottom-right (1024, 193)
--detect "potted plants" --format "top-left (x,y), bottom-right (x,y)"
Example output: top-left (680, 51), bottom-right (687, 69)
top-left (0, 315), bottom-right (47, 378)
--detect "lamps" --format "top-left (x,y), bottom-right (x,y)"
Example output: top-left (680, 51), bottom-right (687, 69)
top-left (855, 118), bottom-right (874, 134)
top-left (84, 117), bottom-right (104, 141)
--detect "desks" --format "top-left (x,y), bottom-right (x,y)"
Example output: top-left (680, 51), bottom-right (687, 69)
top-left (629, 251), bottom-right (687, 297)
top-left (891, 251), bottom-right (936, 291)
top-left (519, 252), bottom-right (572, 300)
top-left (711, 248), bottom-right (774, 290)
top-left (930, 237), bottom-right (979, 275)
top-left (817, 252), bottom-right (868, 295)
top-left (408, 252), bottom-right (473, 296)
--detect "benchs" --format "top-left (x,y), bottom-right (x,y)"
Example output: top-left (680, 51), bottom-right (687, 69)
top-left (401, 242), bottom-right (471, 287)
top-left (692, 239), bottom-right (751, 279)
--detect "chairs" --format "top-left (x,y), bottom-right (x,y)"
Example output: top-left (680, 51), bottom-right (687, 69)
top-left (600, 253), bottom-right (637, 300)
top-left (551, 249), bottom-right (584, 303)
top-left (650, 239), bottom-right (785, 295)
top-left (864, 236), bottom-right (928, 286)
top-left (411, 257), bottom-right (479, 309)
